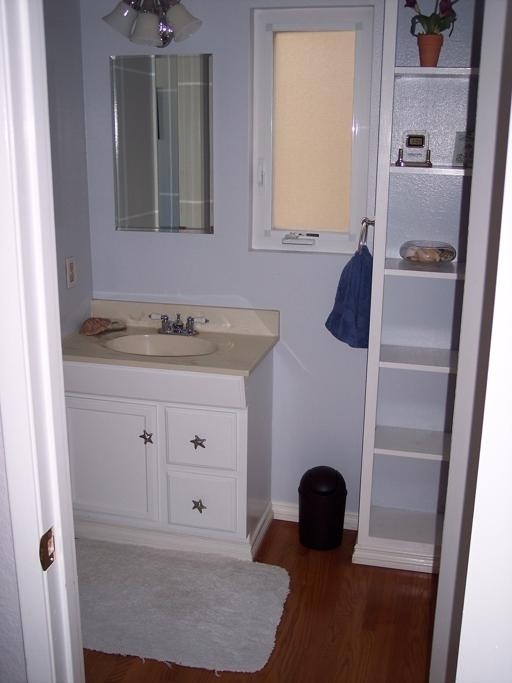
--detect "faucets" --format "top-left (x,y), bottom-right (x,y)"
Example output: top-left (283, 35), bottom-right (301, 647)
top-left (173, 313), bottom-right (183, 335)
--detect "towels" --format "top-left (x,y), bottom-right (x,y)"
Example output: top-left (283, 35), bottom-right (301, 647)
top-left (325, 247), bottom-right (373, 350)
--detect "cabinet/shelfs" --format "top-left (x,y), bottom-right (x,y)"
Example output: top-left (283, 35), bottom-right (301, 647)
top-left (351, 0), bottom-right (506, 575)
top-left (63, 299), bottom-right (283, 562)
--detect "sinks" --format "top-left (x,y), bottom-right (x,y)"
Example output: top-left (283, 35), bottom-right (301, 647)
top-left (97, 329), bottom-right (226, 359)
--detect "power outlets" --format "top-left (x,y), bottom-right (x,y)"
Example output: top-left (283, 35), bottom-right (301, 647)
top-left (67, 261), bottom-right (77, 289)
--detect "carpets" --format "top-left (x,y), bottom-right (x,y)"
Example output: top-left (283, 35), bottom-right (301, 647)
top-left (76, 540), bottom-right (289, 673)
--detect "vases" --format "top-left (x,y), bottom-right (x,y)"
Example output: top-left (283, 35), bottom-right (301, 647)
top-left (416, 34), bottom-right (442, 67)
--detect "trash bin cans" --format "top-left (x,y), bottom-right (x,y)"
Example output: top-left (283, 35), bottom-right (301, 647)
top-left (299, 465), bottom-right (347, 553)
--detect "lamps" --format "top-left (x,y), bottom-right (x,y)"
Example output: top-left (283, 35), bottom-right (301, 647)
top-left (102, 1), bottom-right (202, 48)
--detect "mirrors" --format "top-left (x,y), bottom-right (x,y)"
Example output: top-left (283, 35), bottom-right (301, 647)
top-left (110, 54), bottom-right (215, 234)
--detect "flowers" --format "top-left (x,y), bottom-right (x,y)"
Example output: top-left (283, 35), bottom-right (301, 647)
top-left (402, 0), bottom-right (457, 37)
